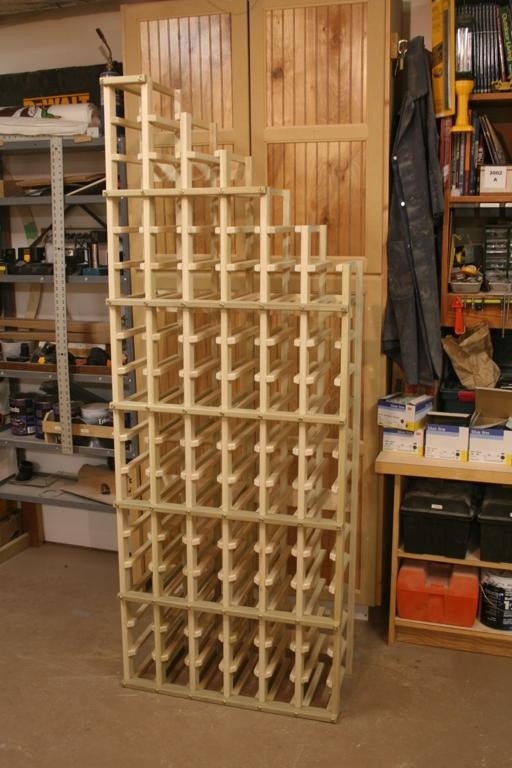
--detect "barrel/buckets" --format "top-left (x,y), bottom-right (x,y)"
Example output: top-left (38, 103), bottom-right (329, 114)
top-left (477, 569), bottom-right (512, 632)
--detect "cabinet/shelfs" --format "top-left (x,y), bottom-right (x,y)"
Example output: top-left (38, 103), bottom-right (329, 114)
top-left (0, 128), bottom-right (116, 556)
top-left (373, 2), bottom-right (511, 661)
top-left (124, 2), bottom-right (384, 609)
